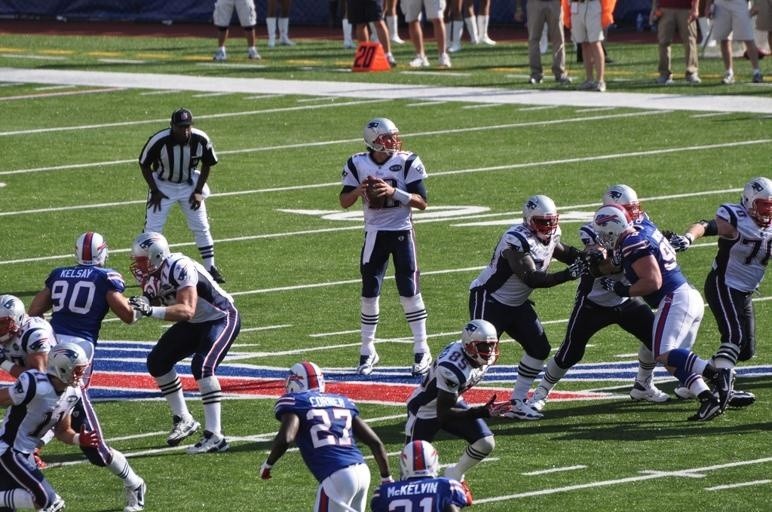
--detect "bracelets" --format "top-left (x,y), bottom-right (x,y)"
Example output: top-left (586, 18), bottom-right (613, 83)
top-left (621, 286), bottom-right (630, 297)
top-left (392, 188), bottom-right (412, 205)
top-left (0, 360), bottom-right (15, 373)
top-left (151, 189), bottom-right (159, 194)
top-left (684, 233), bottom-right (694, 245)
top-left (73, 433), bottom-right (80, 445)
top-left (470, 407), bottom-right (483, 419)
top-left (150, 306), bottom-right (166, 320)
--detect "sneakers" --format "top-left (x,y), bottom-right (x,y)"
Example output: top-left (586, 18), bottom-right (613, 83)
top-left (522, 48), bottom-right (615, 91)
top-left (411, 350), bottom-right (432, 379)
top-left (685, 73), bottom-right (702, 85)
top-left (39, 497), bottom-right (65, 511)
top-left (504, 399), bottom-right (544, 421)
top-left (124, 477), bottom-right (146, 511)
top-left (528, 388), bottom-right (547, 410)
top-left (249, 51), bottom-right (262, 60)
top-left (356, 351), bottom-right (380, 378)
top-left (752, 69), bottom-right (763, 83)
top-left (166, 415), bottom-right (201, 447)
top-left (340, 36), bottom-right (501, 70)
top-left (213, 51), bottom-right (229, 62)
top-left (674, 367), bottom-right (757, 422)
top-left (653, 73), bottom-right (673, 86)
top-left (268, 41), bottom-right (275, 49)
top-left (629, 375), bottom-right (670, 403)
top-left (280, 40), bottom-right (295, 47)
top-left (185, 431), bottom-right (229, 455)
top-left (721, 72), bottom-right (734, 85)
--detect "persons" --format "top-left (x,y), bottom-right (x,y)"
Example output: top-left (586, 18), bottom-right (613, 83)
top-left (127, 232), bottom-right (241, 453)
top-left (593, 204), bottom-right (737, 421)
top-left (28, 230), bottom-right (160, 469)
top-left (139, 108), bottom-right (226, 286)
top-left (339, 117), bottom-right (433, 376)
top-left (370, 440), bottom-right (468, 512)
top-left (404, 319), bottom-right (513, 506)
top-left (0, 343), bottom-right (100, 512)
top-left (0, 294), bottom-right (147, 512)
top-left (260, 361), bottom-right (395, 512)
top-left (468, 194), bottom-right (597, 420)
top-left (528, 184), bottom-right (677, 411)
top-left (670, 177), bottom-right (772, 407)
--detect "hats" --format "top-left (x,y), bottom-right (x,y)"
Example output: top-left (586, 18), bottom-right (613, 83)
top-left (171, 108), bottom-right (192, 127)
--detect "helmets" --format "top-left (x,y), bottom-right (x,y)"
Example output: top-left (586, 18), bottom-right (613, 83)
top-left (129, 232), bottom-right (171, 279)
top-left (284, 360), bottom-right (326, 394)
top-left (741, 176), bottom-right (772, 227)
top-left (398, 439), bottom-right (438, 479)
top-left (47, 342), bottom-right (88, 387)
top-left (521, 195), bottom-right (558, 240)
top-left (361, 115), bottom-right (402, 155)
top-left (602, 184), bottom-right (639, 222)
top-left (74, 231), bottom-right (108, 266)
top-left (1, 294), bottom-right (27, 344)
top-left (461, 319), bottom-right (499, 366)
top-left (592, 204), bottom-right (634, 250)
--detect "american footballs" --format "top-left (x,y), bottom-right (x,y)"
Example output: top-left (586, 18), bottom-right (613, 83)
top-left (366, 175), bottom-right (388, 212)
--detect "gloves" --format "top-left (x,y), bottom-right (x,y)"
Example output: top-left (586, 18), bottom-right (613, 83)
top-left (257, 458), bottom-right (273, 481)
top-left (130, 296), bottom-right (153, 318)
top-left (485, 393), bottom-right (516, 418)
top-left (569, 242), bottom-right (624, 278)
top-left (601, 278), bottom-right (630, 298)
top-left (662, 229), bottom-right (694, 253)
